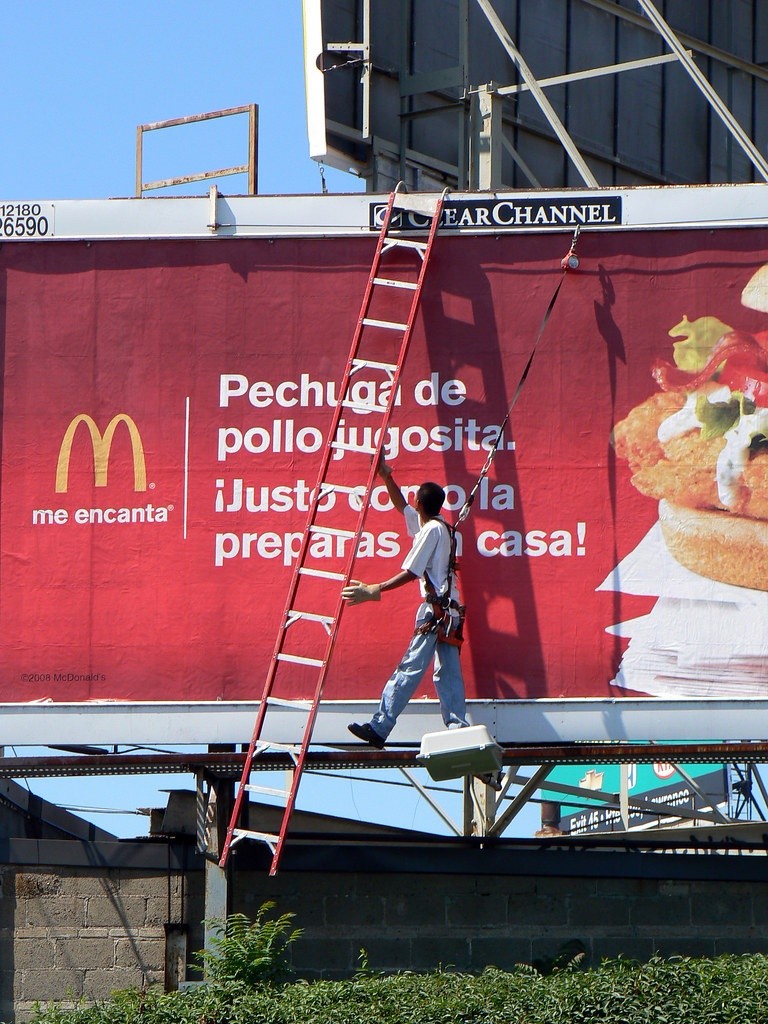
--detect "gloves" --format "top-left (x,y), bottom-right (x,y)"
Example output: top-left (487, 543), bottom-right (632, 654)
top-left (341, 580), bottom-right (381, 606)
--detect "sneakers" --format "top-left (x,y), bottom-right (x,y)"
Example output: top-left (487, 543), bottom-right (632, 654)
top-left (348, 722), bottom-right (385, 750)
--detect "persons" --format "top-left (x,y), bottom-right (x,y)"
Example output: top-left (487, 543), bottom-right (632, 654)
top-left (340, 445), bottom-right (469, 750)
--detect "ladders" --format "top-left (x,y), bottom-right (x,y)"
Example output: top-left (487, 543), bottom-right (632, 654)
top-left (219, 181), bottom-right (455, 877)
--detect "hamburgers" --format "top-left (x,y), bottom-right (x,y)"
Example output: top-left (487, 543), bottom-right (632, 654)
top-left (608, 263), bottom-right (768, 591)
